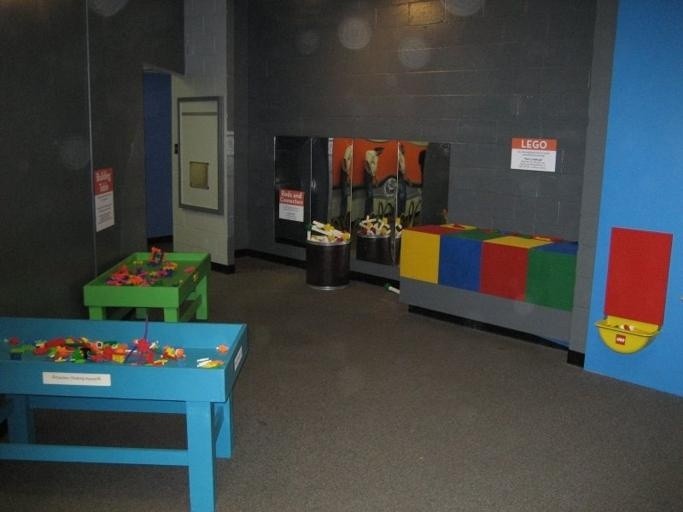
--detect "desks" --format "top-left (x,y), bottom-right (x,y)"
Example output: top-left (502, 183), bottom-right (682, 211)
top-left (400, 225), bottom-right (578, 348)
top-left (0, 317), bottom-right (249, 512)
top-left (83, 251), bottom-right (211, 324)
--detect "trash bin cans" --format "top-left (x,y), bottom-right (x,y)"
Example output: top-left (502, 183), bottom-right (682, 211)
top-left (305, 238), bottom-right (353, 291)
top-left (356, 233), bottom-right (391, 265)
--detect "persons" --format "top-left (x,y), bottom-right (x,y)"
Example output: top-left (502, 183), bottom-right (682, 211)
top-left (341, 146), bottom-right (406, 221)
